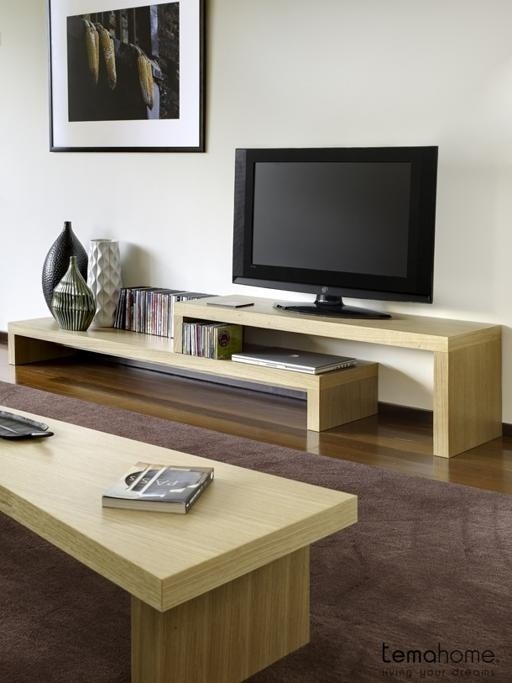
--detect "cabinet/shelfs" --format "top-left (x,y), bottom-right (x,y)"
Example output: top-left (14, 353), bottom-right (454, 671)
top-left (8, 293), bottom-right (502, 463)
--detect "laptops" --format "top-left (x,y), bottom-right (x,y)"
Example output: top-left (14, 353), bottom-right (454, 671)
top-left (231, 348), bottom-right (359, 376)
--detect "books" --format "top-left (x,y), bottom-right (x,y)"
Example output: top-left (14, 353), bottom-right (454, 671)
top-left (113, 286), bottom-right (244, 361)
top-left (102, 461), bottom-right (213, 515)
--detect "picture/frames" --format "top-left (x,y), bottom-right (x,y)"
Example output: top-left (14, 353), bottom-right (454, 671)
top-left (48, 0), bottom-right (207, 153)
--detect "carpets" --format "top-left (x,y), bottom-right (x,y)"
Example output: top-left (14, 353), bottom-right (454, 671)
top-left (1, 381), bottom-right (509, 681)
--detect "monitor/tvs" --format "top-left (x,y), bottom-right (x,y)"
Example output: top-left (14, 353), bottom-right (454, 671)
top-left (232, 145), bottom-right (439, 321)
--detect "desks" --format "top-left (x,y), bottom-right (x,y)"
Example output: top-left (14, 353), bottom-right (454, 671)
top-left (0, 406), bottom-right (358, 682)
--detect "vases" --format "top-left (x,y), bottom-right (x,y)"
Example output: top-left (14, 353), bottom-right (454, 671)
top-left (86, 237), bottom-right (124, 327)
top-left (41, 219), bottom-right (89, 318)
top-left (52, 255), bottom-right (97, 331)
